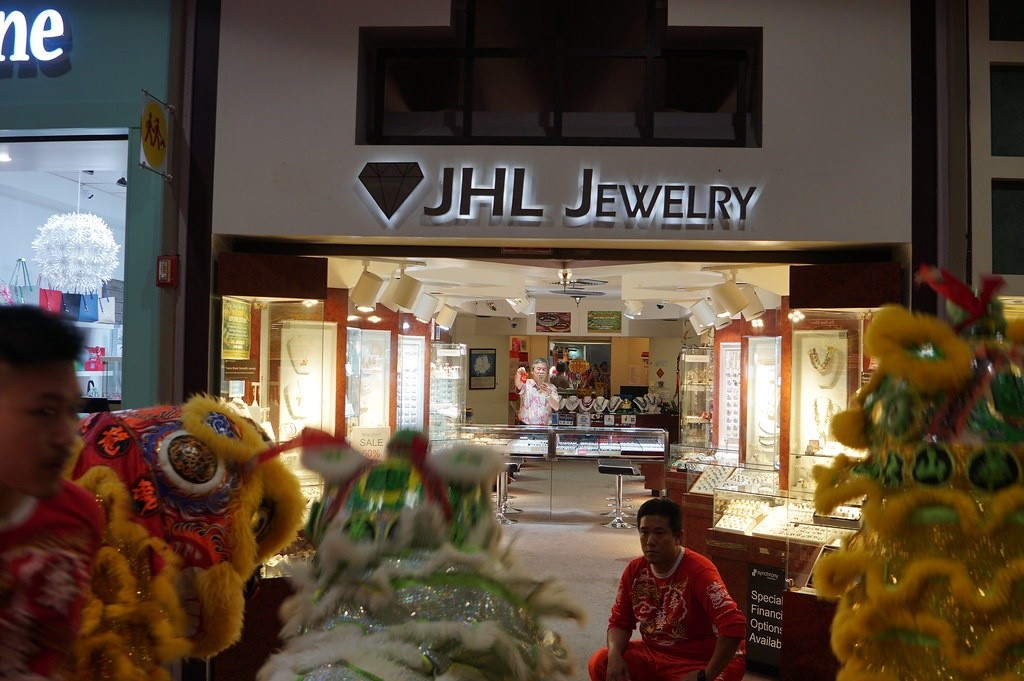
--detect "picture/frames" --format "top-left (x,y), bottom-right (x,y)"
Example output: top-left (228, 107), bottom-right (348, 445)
top-left (469, 348), bottom-right (496, 390)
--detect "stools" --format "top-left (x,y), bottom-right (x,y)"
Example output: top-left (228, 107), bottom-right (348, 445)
top-left (596, 464), bottom-right (639, 527)
top-left (598, 458), bottom-right (633, 518)
top-left (490, 463), bottom-right (520, 524)
top-left (491, 463), bottom-right (522, 513)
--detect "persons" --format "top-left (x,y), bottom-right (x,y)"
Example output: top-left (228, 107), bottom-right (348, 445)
top-left (587, 362), bottom-right (610, 399)
top-left (550, 361), bottom-right (571, 388)
top-left (514, 358), bottom-right (559, 426)
top-left (595, 498), bottom-right (753, 681)
top-left (0, 303), bottom-right (103, 681)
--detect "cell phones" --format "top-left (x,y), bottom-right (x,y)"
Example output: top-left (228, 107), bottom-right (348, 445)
top-left (538, 374), bottom-right (545, 386)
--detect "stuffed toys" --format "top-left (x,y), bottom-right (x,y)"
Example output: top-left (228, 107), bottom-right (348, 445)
top-left (814, 267), bottom-right (1024, 681)
top-left (257, 426), bottom-right (587, 681)
top-left (62, 396), bottom-right (302, 681)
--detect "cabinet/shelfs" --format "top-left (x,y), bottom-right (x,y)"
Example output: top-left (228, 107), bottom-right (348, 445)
top-left (668, 443), bottom-right (863, 681)
top-left (680, 345), bottom-right (715, 450)
top-left (58, 319), bottom-right (123, 412)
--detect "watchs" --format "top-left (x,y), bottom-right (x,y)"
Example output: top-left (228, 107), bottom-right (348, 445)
top-left (697, 669), bottom-right (706, 681)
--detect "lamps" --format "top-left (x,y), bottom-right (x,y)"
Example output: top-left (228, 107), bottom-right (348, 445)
top-left (507, 298), bottom-right (536, 316)
top-left (351, 261), bottom-right (458, 332)
top-left (80, 169), bottom-right (127, 199)
top-left (624, 301), bottom-right (644, 318)
top-left (686, 266), bottom-right (765, 336)
